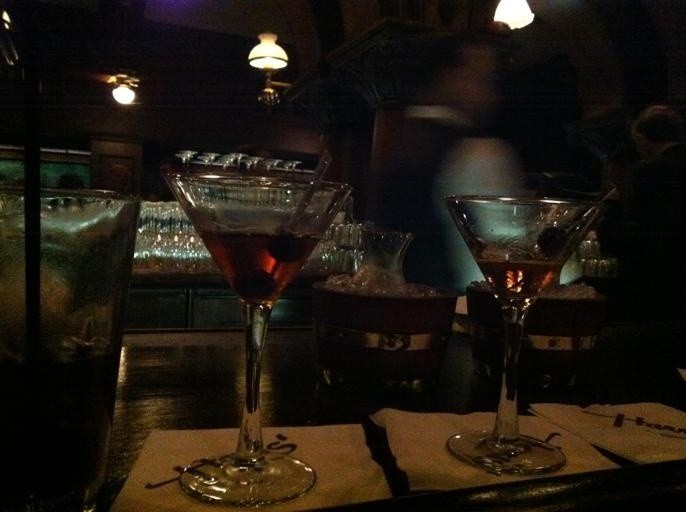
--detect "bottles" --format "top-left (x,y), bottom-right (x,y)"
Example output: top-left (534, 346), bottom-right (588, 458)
top-left (354, 223), bottom-right (416, 289)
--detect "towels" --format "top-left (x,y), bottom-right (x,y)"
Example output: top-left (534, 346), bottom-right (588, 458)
top-left (371, 409), bottom-right (623, 492)
top-left (529, 402), bottom-right (686, 465)
top-left (112, 422), bottom-right (392, 511)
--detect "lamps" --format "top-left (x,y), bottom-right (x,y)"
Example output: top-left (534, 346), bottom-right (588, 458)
top-left (107, 70), bottom-right (141, 105)
top-left (247, 31), bottom-right (292, 105)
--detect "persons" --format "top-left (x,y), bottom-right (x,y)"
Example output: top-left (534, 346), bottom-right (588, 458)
top-left (367, 39), bottom-right (592, 412)
top-left (620, 102), bottom-right (686, 416)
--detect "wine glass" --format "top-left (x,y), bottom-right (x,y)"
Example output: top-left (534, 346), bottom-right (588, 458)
top-left (173, 150), bottom-right (303, 169)
top-left (443, 194), bottom-right (604, 476)
top-left (162, 169), bottom-right (357, 509)
